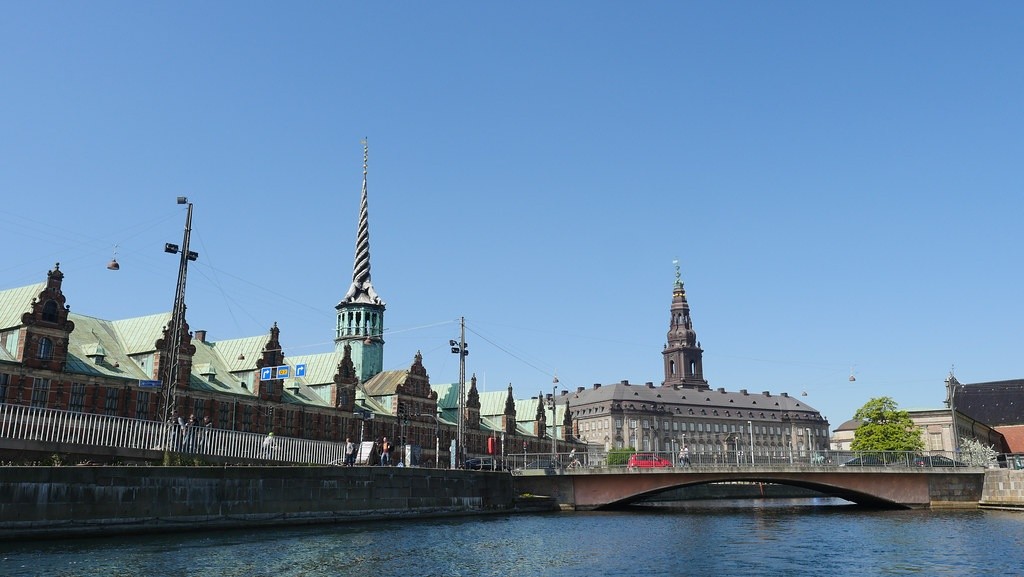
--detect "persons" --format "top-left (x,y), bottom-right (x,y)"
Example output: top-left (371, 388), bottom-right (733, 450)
top-left (569, 447), bottom-right (577, 465)
top-left (165, 410), bottom-right (213, 454)
top-left (345, 438), bottom-right (353, 467)
top-left (381, 437), bottom-right (391, 467)
top-left (678, 446), bottom-right (693, 469)
top-left (261, 432), bottom-right (279, 458)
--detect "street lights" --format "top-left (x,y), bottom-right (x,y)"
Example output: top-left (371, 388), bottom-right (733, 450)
top-left (806, 428), bottom-right (812, 466)
top-left (748, 421), bottom-right (754, 467)
top-left (635, 428), bottom-right (638, 455)
top-left (735, 437), bottom-right (739, 467)
top-left (682, 434), bottom-right (685, 448)
top-left (421, 414), bottom-right (439, 468)
top-left (353, 413), bottom-right (364, 466)
top-left (788, 441), bottom-right (793, 465)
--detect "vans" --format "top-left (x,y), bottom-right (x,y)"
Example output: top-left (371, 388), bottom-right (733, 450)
top-left (628, 454), bottom-right (673, 467)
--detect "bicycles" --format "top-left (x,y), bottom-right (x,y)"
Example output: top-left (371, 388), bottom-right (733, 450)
top-left (565, 457), bottom-right (582, 469)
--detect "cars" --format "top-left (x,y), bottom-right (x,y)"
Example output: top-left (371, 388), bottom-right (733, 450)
top-left (527, 460), bottom-right (562, 469)
top-left (466, 459), bottom-right (501, 471)
top-left (917, 456), bottom-right (965, 467)
top-left (846, 456), bottom-right (883, 464)
